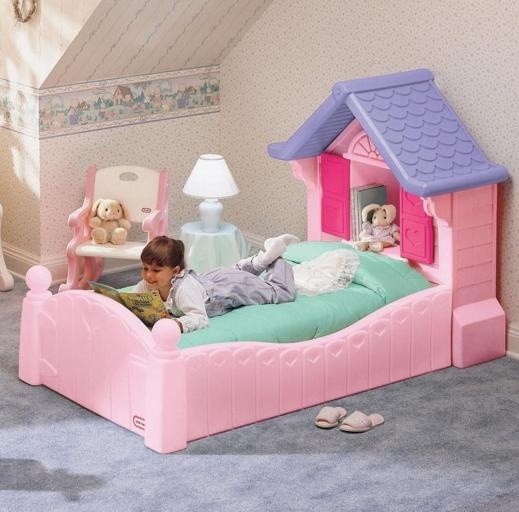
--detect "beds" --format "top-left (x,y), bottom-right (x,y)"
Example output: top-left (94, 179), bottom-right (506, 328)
top-left (17, 242), bottom-right (454, 454)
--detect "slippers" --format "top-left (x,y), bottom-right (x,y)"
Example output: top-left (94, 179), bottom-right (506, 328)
top-left (316, 406), bottom-right (347, 429)
top-left (340, 411), bottom-right (384, 433)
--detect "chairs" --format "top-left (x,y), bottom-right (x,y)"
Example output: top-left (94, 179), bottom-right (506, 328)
top-left (58, 164), bottom-right (171, 293)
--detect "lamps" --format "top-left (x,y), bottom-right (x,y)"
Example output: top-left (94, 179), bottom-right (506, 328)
top-left (182, 152), bottom-right (242, 233)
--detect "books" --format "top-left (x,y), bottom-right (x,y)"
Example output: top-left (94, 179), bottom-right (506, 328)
top-left (86, 280), bottom-right (169, 327)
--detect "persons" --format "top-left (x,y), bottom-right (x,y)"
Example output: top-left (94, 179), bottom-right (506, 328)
top-left (131, 234), bottom-right (301, 332)
